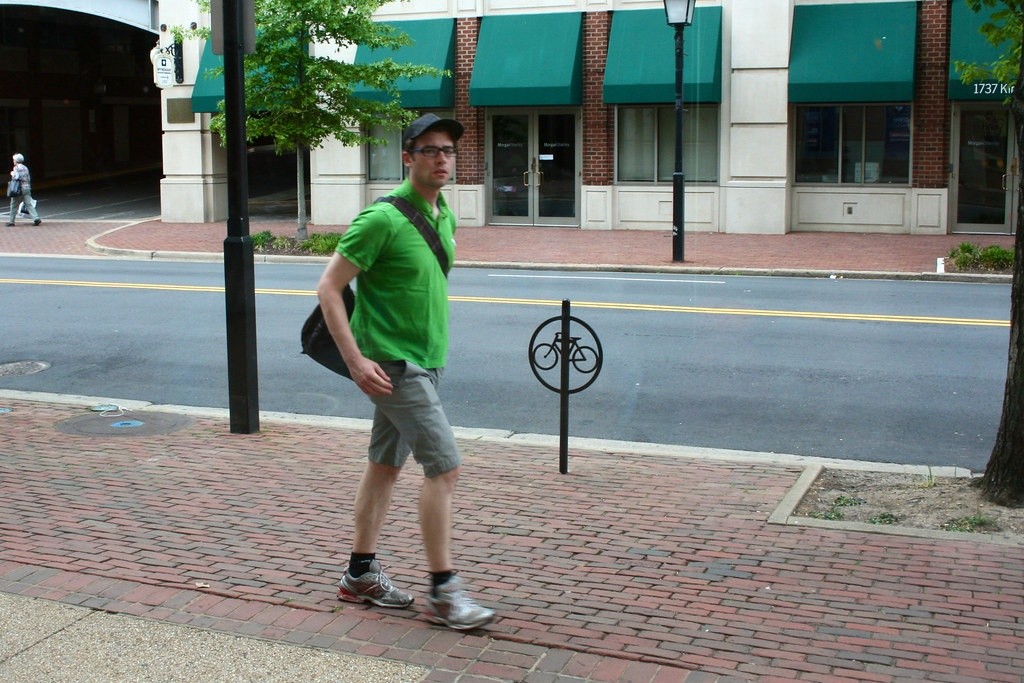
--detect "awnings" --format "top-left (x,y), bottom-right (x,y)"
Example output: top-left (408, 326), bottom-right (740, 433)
top-left (602, 7), bottom-right (722, 104)
top-left (469, 12), bottom-right (584, 106)
top-left (948, 0), bottom-right (1024, 99)
top-left (349, 19), bottom-right (455, 108)
top-left (788, 1), bottom-right (918, 103)
top-left (191, 25), bottom-right (308, 112)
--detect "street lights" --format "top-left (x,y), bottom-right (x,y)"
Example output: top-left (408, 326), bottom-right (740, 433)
top-left (661, 0), bottom-right (698, 265)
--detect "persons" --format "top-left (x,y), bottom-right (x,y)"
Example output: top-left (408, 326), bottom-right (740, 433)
top-left (5, 153), bottom-right (41, 226)
top-left (317, 112), bottom-right (496, 631)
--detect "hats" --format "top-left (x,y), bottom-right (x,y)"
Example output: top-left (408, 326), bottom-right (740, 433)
top-left (402, 112), bottom-right (465, 139)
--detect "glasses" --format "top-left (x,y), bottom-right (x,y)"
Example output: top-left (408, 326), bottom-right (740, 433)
top-left (409, 146), bottom-right (460, 157)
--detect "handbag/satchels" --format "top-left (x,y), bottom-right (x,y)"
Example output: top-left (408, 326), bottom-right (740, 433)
top-left (18, 196), bottom-right (37, 218)
top-left (7, 179), bottom-right (22, 197)
top-left (296, 282), bottom-right (359, 382)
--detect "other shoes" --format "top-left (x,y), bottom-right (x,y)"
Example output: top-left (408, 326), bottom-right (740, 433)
top-left (5, 222), bottom-right (15, 227)
top-left (32, 219), bottom-right (42, 226)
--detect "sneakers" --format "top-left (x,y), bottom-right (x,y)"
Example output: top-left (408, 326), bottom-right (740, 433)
top-left (332, 560), bottom-right (416, 608)
top-left (420, 575), bottom-right (497, 632)
